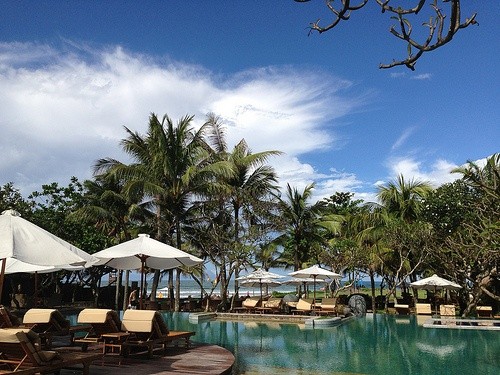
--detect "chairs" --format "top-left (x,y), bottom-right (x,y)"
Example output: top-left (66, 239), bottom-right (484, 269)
top-left (392, 303), bottom-right (494, 318)
top-left (0, 304), bottom-right (196, 375)
top-left (240, 296), bottom-right (340, 318)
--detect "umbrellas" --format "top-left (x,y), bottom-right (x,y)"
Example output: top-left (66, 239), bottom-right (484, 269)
top-left (91, 234), bottom-right (203, 310)
top-left (410, 274), bottom-right (463, 314)
top-left (0, 210), bottom-right (101, 301)
top-left (237, 265), bottom-right (341, 309)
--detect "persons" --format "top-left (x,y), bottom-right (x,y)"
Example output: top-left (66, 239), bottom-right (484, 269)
top-left (129, 289), bottom-right (138, 309)
